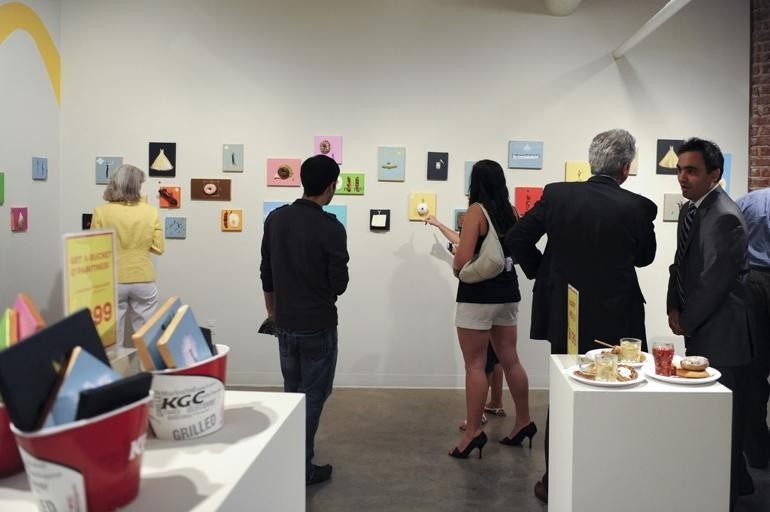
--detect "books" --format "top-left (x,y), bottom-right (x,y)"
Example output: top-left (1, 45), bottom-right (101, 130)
top-left (1, 293), bottom-right (216, 434)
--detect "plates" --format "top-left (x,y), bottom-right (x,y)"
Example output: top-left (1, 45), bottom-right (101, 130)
top-left (643, 358), bottom-right (723, 386)
top-left (584, 347), bottom-right (654, 371)
top-left (569, 366), bottom-right (646, 388)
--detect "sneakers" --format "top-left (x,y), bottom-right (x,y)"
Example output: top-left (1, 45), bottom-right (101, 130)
top-left (306, 463), bottom-right (331, 486)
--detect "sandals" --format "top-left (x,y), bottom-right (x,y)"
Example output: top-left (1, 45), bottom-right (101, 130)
top-left (457, 413), bottom-right (487, 431)
top-left (483, 404), bottom-right (506, 416)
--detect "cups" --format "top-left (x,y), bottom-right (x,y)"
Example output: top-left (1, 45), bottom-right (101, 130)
top-left (651, 342), bottom-right (675, 377)
top-left (621, 337), bottom-right (642, 365)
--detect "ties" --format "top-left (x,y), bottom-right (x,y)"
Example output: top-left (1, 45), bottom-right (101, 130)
top-left (675, 204), bottom-right (697, 306)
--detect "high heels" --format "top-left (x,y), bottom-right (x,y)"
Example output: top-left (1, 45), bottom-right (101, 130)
top-left (498, 420), bottom-right (537, 448)
top-left (448, 430), bottom-right (487, 459)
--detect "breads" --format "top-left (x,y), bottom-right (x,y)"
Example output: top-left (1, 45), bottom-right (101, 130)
top-left (615, 364), bottom-right (638, 382)
top-left (674, 368), bottom-right (708, 378)
top-left (680, 356), bottom-right (710, 371)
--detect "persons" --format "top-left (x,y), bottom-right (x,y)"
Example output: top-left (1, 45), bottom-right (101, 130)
top-left (425, 201), bottom-right (506, 432)
top-left (423, 158), bottom-right (538, 461)
top-left (259, 152), bottom-right (354, 488)
top-left (505, 127), bottom-right (658, 507)
top-left (88, 161), bottom-right (166, 347)
top-left (734, 185), bottom-right (770, 473)
top-left (663, 135), bottom-right (753, 511)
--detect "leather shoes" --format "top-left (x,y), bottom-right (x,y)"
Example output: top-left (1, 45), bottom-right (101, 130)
top-left (535, 481), bottom-right (546, 502)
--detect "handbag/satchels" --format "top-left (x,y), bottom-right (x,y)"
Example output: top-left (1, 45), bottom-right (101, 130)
top-left (459, 228), bottom-right (506, 284)
